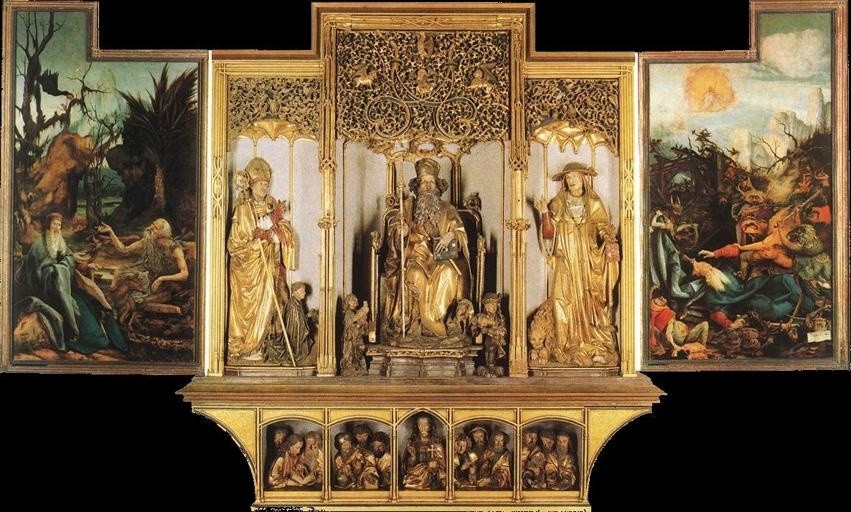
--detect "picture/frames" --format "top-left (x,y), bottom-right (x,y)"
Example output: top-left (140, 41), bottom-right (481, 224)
top-left (2, 0), bottom-right (851, 383)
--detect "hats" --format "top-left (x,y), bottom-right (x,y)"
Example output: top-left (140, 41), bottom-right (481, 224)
top-left (47, 213), bottom-right (63, 221)
top-left (415, 158), bottom-right (440, 178)
top-left (245, 158), bottom-right (272, 182)
top-left (483, 292), bottom-right (503, 303)
top-left (552, 162), bottom-right (598, 181)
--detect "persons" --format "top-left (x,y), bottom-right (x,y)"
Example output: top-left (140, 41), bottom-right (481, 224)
top-left (381, 158), bottom-right (473, 348)
top-left (523, 428), bottom-right (584, 492)
top-left (528, 161), bottom-right (620, 365)
top-left (453, 426), bottom-right (513, 490)
top-left (226, 157), bottom-right (319, 360)
top-left (332, 425), bottom-right (392, 489)
top-left (401, 413), bottom-right (449, 494)
top-left (266, 427), bottom-right (325, 488)
top-left (16, 211), bottom-right (190, 344)
top-left (648, 201), bottom-right (836, 357)
